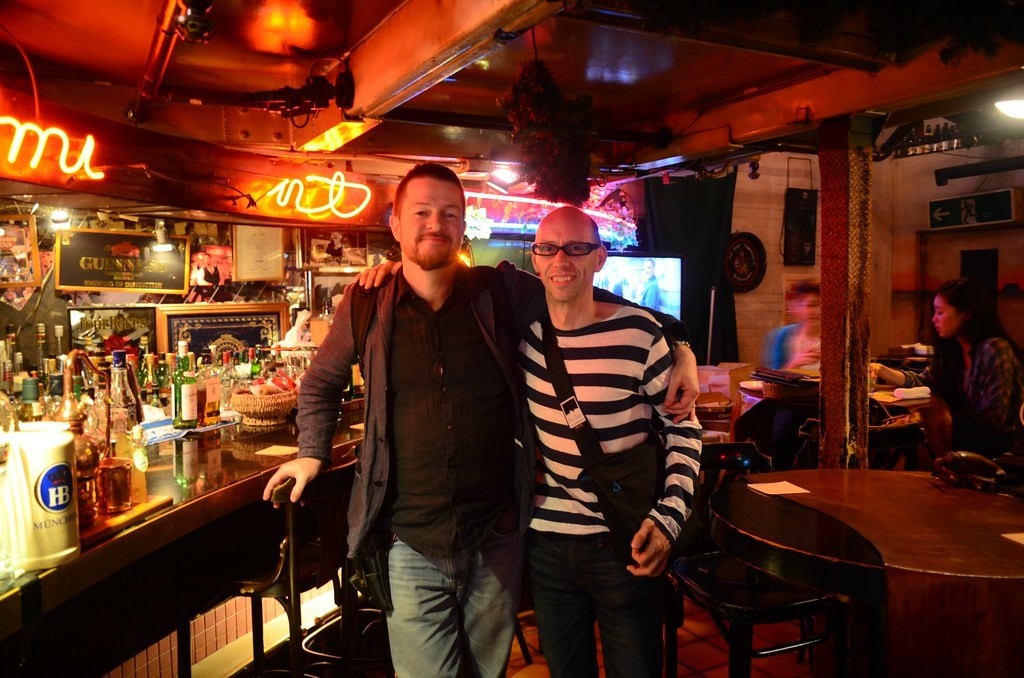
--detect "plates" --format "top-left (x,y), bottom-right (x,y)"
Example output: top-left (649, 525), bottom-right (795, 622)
top-left (739, 380), bottom-right (763, 392)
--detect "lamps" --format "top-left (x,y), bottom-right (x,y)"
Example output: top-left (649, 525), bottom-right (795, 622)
top-left (154, 219), bottom-right (172, 253)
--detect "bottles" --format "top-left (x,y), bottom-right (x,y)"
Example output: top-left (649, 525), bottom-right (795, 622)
top-left (894, 118), bottom-right (995, 157)
top-left (197, 428), bottom-right (225, 491)
top-left (171, 436), bottom-right (200, 501)
top-left (0, 318), bottom-right (352, 534)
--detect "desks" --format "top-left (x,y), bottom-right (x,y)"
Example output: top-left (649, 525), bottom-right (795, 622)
top-left (729, 381), bottom-right (943, 471)
top-left (708, 469), bottom-right (1022, 678)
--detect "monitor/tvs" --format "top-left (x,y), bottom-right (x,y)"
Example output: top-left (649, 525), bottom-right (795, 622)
top-left (593, 251), bottom-right (685, 322)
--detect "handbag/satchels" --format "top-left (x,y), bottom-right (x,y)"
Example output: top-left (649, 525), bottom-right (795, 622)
top-left (577, 433), bottom-right (708, 562)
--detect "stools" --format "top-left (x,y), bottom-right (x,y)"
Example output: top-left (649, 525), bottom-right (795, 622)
top-left (174, 465), bottom-right (353, 678)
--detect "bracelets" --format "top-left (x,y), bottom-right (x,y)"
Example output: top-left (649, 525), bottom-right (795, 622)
top-left (672, 340), bottom-right (691, 349)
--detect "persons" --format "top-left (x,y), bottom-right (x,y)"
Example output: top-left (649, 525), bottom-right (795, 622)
top-left (352, 204), bottom-right (703, 678)
top-left (771, 286), bottom-right (821, 453)
top-left (191, 254), bottom-right (220, 286)
top-left (867, 277), bottom-right (1024, 496)
top-left (312, 232), bottom-right (346, 262)
top-left (264, 162), bottom-right (699, 678)
top-left (613, 258), bottom-right (660, 312)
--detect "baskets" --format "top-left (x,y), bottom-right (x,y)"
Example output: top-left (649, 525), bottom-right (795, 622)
top-left (228, 384), bottom-right (300, 427)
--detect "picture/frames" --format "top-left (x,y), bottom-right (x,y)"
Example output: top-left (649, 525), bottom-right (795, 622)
top-left (308, 270), bottom-right (361, 318)
top-left (156, 302), bottom-right (289, 366)
top-left (0, 215), bottom-right (42, 289)
top-left (298, 227), bottom-right (369, 268)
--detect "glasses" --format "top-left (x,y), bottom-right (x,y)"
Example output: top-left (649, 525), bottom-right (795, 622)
top-left (530, 241), bottom-right (601, 256)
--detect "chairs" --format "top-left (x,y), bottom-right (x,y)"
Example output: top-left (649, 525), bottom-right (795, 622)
top-left (666, 426), bottom-right (923, 678)
